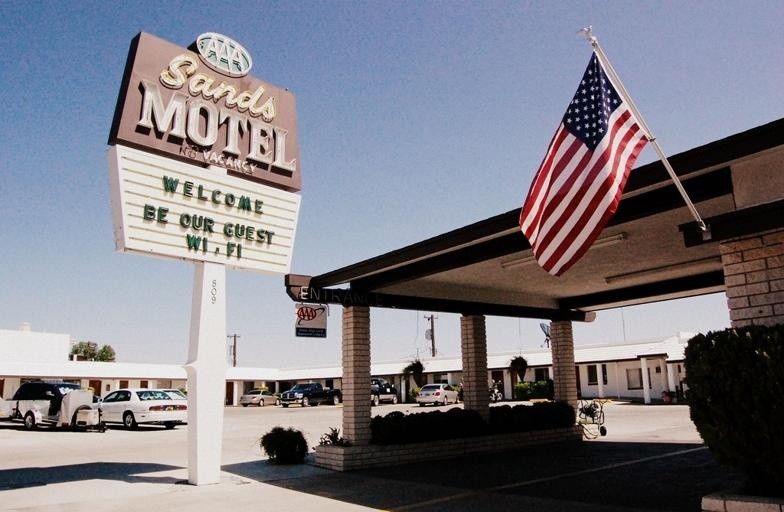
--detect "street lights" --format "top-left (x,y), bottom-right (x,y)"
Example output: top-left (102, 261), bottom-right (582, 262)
top-left (227, 333), bottom-right (241, 367)
top-left (422, 310), bottom-right (441, 358)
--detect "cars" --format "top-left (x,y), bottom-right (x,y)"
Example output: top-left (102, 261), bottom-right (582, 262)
top-left (159, 387), bottom-right (189, 428)
top-left (87, 386), bottom-right (190, 431)
top-left (0, 378), bottom-right (103, 433)
top-left (416, 384), bottom-right (461, 407)
top-left (238, 387), bottom-right (281, 409)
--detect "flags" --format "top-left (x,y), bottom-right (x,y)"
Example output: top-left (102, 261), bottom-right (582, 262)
top-left (516, 52), bottom-right (649, 277)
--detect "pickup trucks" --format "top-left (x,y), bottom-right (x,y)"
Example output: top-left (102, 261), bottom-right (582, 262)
top-left (369, 376), bottom-right (399, 406)
top-left (279, 381), bottom-right (344, 409)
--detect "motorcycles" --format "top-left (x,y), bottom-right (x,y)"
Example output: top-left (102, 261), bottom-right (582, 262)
top-left (487, 379), bottom-right (505, 403)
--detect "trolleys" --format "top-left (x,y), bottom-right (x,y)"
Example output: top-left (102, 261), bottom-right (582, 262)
top-left (576, 396), bottom-right (611, 437)
